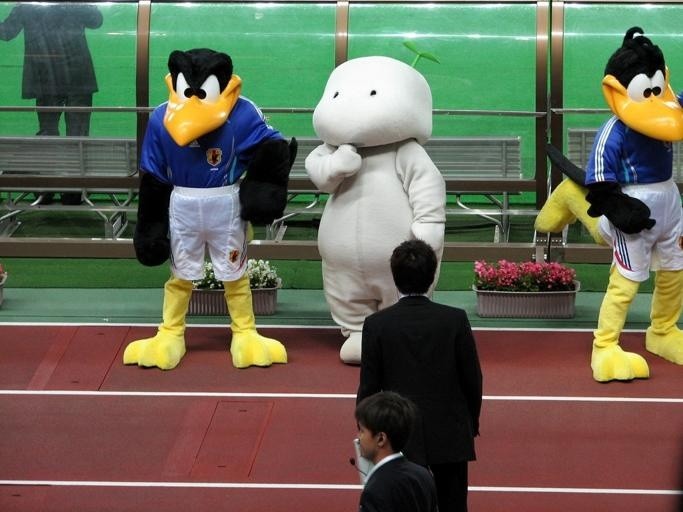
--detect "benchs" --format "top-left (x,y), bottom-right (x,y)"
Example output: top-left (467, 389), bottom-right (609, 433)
top-left (562, 129), bottom-right (683, 244)
top-left (0, 137), bottom-right (139, 239)
top-left (239, 137), bottom-right (522, 243)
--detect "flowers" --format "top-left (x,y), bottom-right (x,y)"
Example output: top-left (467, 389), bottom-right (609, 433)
top-left (193, 259), bottom-right (277, 288)
top-left (474, 259), bottom-right (575, 291)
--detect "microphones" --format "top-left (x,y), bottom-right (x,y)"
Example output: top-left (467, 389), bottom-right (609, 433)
top-left (349, 457), bottom-right (370, 477)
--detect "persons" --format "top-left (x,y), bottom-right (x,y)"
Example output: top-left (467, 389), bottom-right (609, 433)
top-left (0, 2), bottom-right (102, 205)
top-left (356, 239), bottom-right (483, 512)
top-left (355, 390), bottom-right (439, 512)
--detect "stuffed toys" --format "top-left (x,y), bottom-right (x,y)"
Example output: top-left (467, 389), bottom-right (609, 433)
top-left (533, 26), bottom-right (683, 382)
top-left (123, 48), bottom-right (298, 370)
top-left (305, 43), bottom-right (447, 365)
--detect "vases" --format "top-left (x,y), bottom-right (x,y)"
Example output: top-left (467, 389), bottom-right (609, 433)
top-left (0, 273), bottom-right (7, 306)
top-left (472, 280), bottom-right (580, 317)
top-left (187, 279), bottom-right (281, 316)
